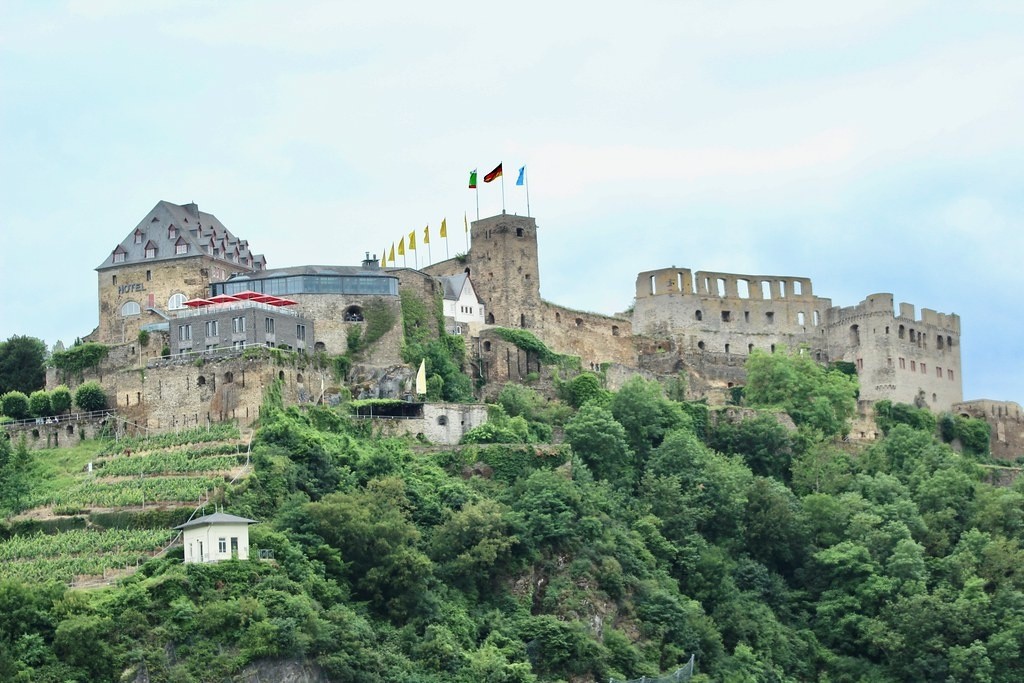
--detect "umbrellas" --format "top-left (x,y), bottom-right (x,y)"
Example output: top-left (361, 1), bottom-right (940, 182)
top-left (181, 290), bottom-right (299, 307)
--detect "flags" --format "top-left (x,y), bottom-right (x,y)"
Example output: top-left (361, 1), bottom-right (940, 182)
top-left (516, 165), bottom-right (525, 185)
top-left (440, 218), bottom-right (447, 237)
top-left (416, 358), bottom-right (427, 394)
top-left (424, 226), bottom-right (429, 243)
top-left (388, 242), bottom-right (395, 261)
top-left (464, 214), bottom-right (468, 233)
top-left (398, 236), bottom-right (405, 255)
top-left (381, 250), bottom-right (386, 267)
top-left (408, 230), bottom-right (416, 249)
top-left (484, 163), bottom-right (503, 183)
top-left (469, 168), bottom-right (477, 188)
